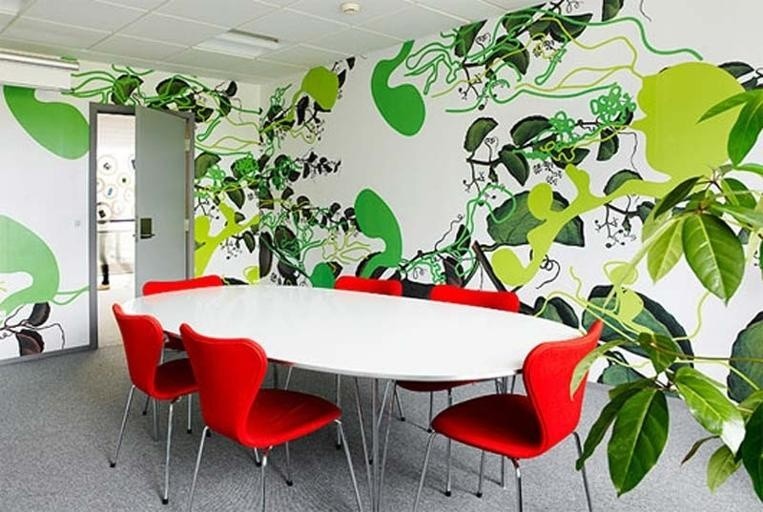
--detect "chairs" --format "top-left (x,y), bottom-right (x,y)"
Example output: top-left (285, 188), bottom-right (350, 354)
top-left (180, 324), bottom-right (362, 510)
top-left (414, 318), bottom-right (603, 511)
top-left (140, 275), bottom-right (227, 434)
top-left (281, 277), bottom-right (408, 450)
top-left (111, 302), bottom-right (261, 504)
top-left (369, 282), bottom-right (520, 497)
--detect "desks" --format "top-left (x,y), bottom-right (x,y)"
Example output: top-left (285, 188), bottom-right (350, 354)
top-left (119, 284), bottom-right (585, 512)
